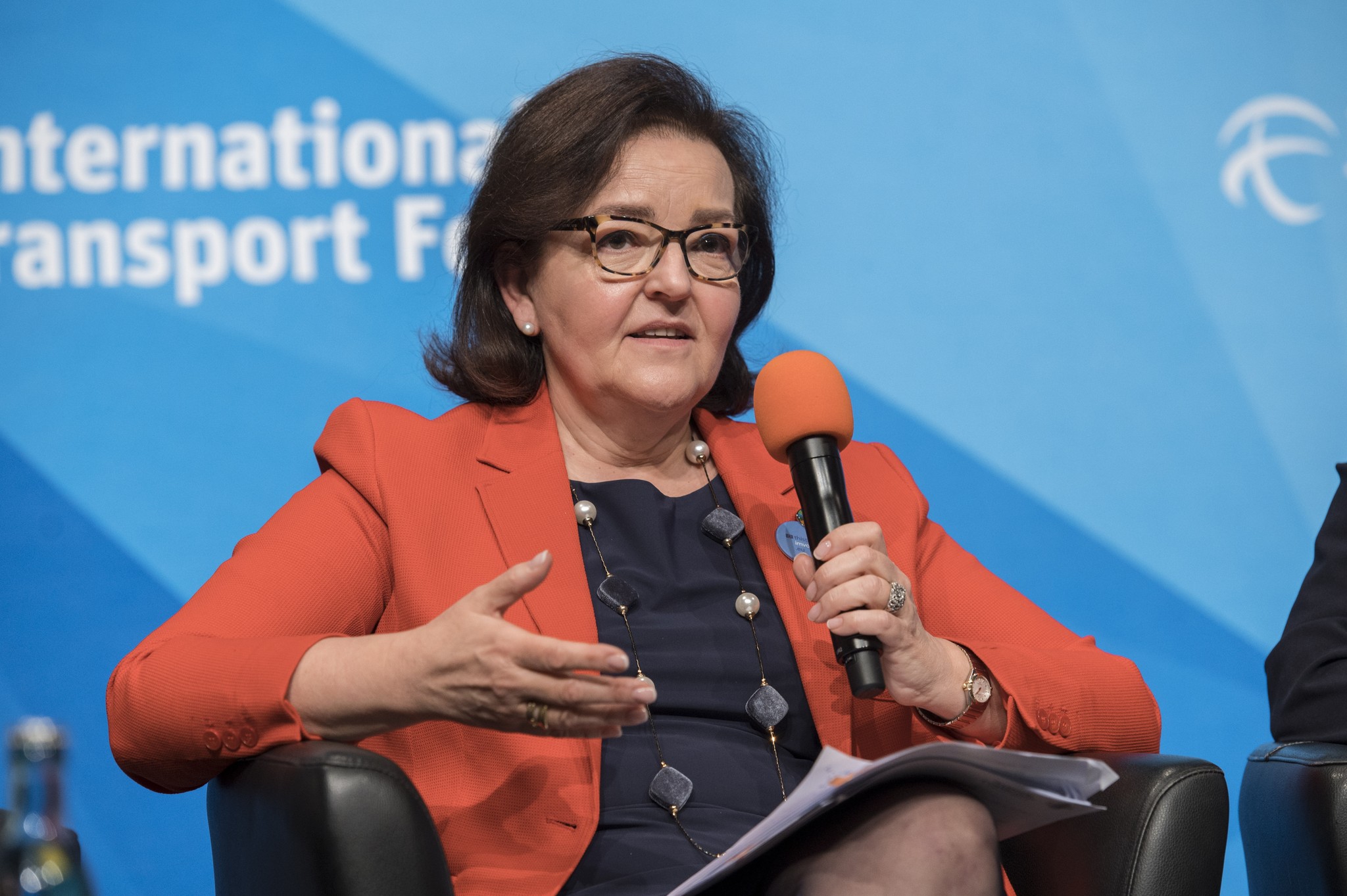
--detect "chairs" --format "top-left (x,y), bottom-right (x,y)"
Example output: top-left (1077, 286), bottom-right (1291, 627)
top-left (204, 738), bottom-right (1231, 896)
top-left (1237, 740), bottom-right (1347, 896)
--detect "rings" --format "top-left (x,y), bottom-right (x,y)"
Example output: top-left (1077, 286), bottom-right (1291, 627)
top-left (526, 702), bottom-right (550, 730)
top-left (883, 582), bottom-right (907, 614)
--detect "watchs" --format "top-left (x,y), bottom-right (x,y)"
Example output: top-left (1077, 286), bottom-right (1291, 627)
top-left (915, 640), bottom-right (993, 731)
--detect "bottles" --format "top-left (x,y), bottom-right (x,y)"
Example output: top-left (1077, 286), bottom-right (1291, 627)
top-left (0, 715), bottom-right (88, 896)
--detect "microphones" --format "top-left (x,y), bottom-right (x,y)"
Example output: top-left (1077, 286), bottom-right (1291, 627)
top-left (754, 351), bottom-right (887, 699)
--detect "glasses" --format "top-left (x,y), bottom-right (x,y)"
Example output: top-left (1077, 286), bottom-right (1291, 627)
top-left (547, 208), bottom-right (751, 282)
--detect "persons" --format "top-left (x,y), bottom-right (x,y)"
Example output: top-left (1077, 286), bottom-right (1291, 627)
top-left (104, 53), bottom-right (1163, 896)
top-left (1264, 462), bottom-right (1347, 748)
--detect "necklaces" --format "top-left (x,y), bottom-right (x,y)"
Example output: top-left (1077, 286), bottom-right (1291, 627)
top-left (569, 436), bottom-right (790, 859)
